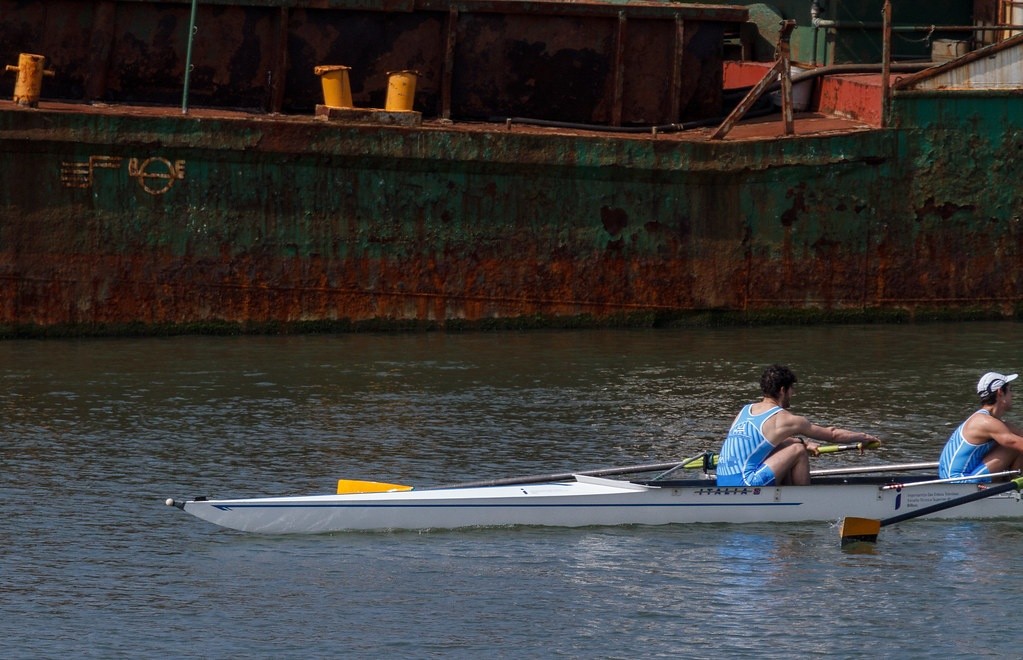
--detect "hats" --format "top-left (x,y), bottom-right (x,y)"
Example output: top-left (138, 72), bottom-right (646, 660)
top-left (977, 372), bottom-right (1018, 398)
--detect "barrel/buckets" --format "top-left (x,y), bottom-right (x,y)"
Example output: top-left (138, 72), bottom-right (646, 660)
top-left (773, 61), bottom-right (817, 112)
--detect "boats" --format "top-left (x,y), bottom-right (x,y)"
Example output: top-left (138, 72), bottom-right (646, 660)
top-left (162, 471), bottom-right (1022, 538)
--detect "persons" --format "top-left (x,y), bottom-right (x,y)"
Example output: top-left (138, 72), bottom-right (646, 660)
top-left (716, 365), bottom-right (881, 486)
top-left (939, 372), bottom-right (1023, 484)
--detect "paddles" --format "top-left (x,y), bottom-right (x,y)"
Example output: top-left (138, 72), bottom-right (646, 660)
top-left (337, 442), bottom-right (866, 495)
top-left (838, 474), bottom-right (1022, 545)
top-left (808, 458), bottom-right (939, 475)
